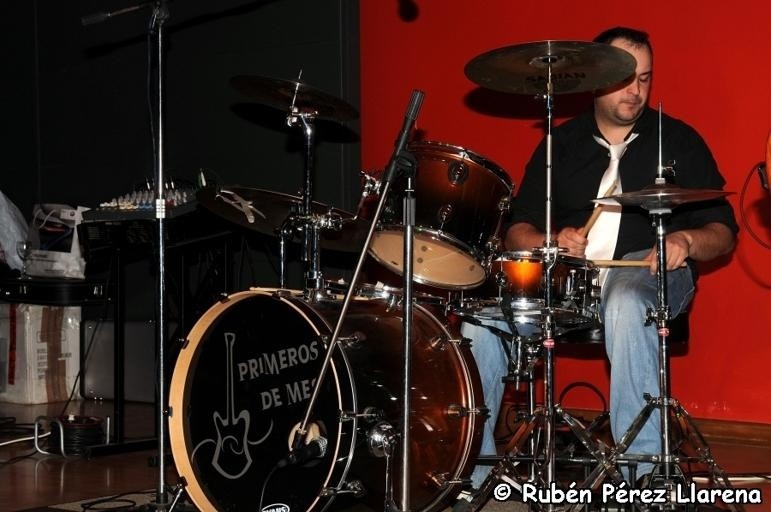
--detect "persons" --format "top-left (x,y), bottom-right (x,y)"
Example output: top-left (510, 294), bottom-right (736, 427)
top-left (456, 27), bottom-right (741, 503)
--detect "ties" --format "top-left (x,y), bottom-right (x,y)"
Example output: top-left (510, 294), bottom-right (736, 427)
top-left (583, 133), bottom-right (638, 297)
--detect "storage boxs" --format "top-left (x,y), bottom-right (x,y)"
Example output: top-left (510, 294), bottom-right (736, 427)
top-left (0, 301), bottom-right (87, 406)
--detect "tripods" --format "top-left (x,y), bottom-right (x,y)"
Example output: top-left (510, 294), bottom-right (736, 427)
top-left (566, 210), bottom-right (744, 512)
top-left (467, 59), bottom-right (648, 512)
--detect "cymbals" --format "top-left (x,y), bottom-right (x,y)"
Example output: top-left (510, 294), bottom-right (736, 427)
top-left (224, 73), bottom-right (359, 120)
top-left (465, 39), bottom-right (638, 95)
top-left (213, 186), bottom-right (372, 251)
top-left (588, 184), bottom-right (736, 208)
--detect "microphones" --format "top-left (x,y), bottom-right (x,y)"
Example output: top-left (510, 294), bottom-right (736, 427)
top-left (278, 435), bottom-right (329, 469)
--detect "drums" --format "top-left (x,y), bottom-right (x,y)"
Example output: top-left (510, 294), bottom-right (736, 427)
top-left (169, 252), bottom-right (597, 511)
top-left (366, 141), bottom-right (514, 290)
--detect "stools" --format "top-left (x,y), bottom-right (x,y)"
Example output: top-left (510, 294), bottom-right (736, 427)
top-left (544, 304), bottom-right (770, 501)
